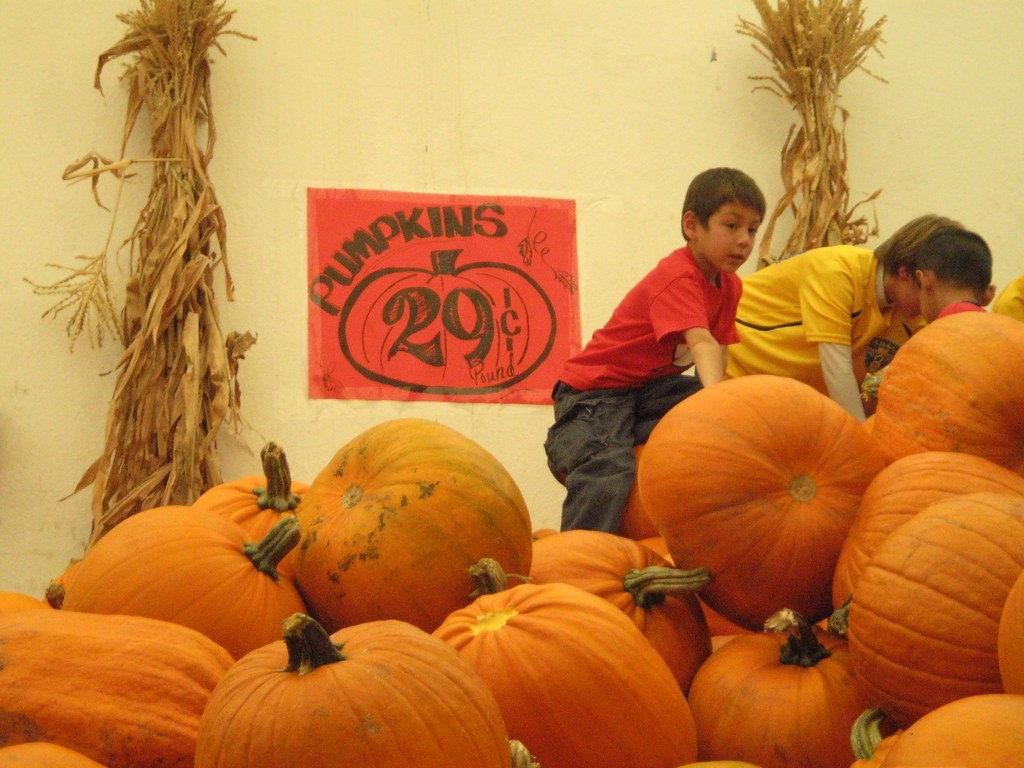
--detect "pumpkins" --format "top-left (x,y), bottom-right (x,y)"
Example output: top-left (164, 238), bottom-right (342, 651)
top-left (1, 310), bottom-right (1024, 767)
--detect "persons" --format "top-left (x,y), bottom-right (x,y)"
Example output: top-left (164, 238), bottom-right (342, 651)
top-left (718, 215), bottom-right (962, 433)
top-left (543, 167), bottom-right (767, 545)
top-left (861, 229), bottom-right (997, 409)
